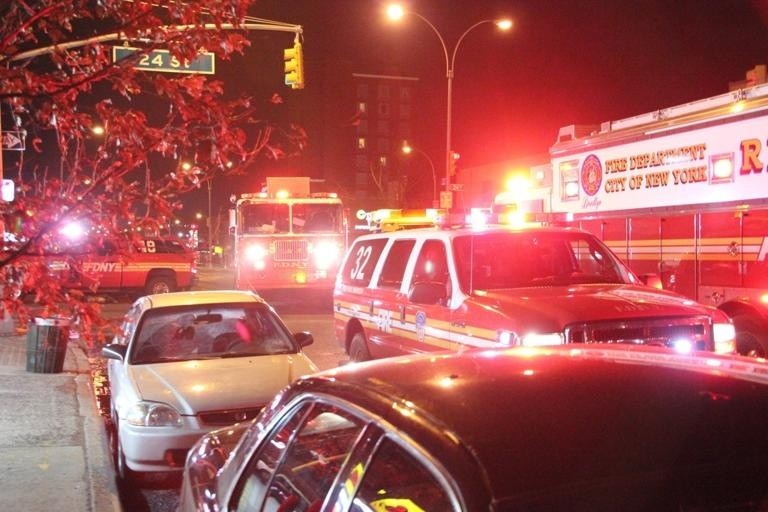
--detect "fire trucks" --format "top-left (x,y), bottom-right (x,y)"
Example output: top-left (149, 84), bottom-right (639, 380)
top-left (228, 174), bottom-right (351, 308)
top-left (542, 62), bottom-right (768, 364)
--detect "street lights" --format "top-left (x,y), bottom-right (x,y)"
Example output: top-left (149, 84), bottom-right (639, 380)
top-left (385, 5), bottom-right (515, 210)
top-left (400, 145), bottom-right (438, 208)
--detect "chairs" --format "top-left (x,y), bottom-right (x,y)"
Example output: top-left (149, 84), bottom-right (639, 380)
top-left (212, 332), bottom-right (239, 352)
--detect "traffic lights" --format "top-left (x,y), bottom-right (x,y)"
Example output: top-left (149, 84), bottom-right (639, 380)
top-left (282, 42), bottom-right (306, 91)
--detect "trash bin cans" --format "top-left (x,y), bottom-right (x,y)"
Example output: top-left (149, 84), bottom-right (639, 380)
top-left (26, 317), bottom-right (72, 373)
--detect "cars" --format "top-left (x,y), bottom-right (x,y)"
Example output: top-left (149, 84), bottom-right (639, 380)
top-left (101, 287), bottom-right (325, 484)
top-left (173, 343), bottom-right (767, 511)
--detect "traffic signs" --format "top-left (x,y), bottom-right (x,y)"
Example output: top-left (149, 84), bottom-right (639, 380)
top-left (112, 46), bottom-right (215, 77)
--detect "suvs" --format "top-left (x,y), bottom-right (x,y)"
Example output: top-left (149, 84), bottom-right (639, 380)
top-left (329, 204), bottom-right (738, 365)
top-left (0, 213), bottom-right (196, 303)
